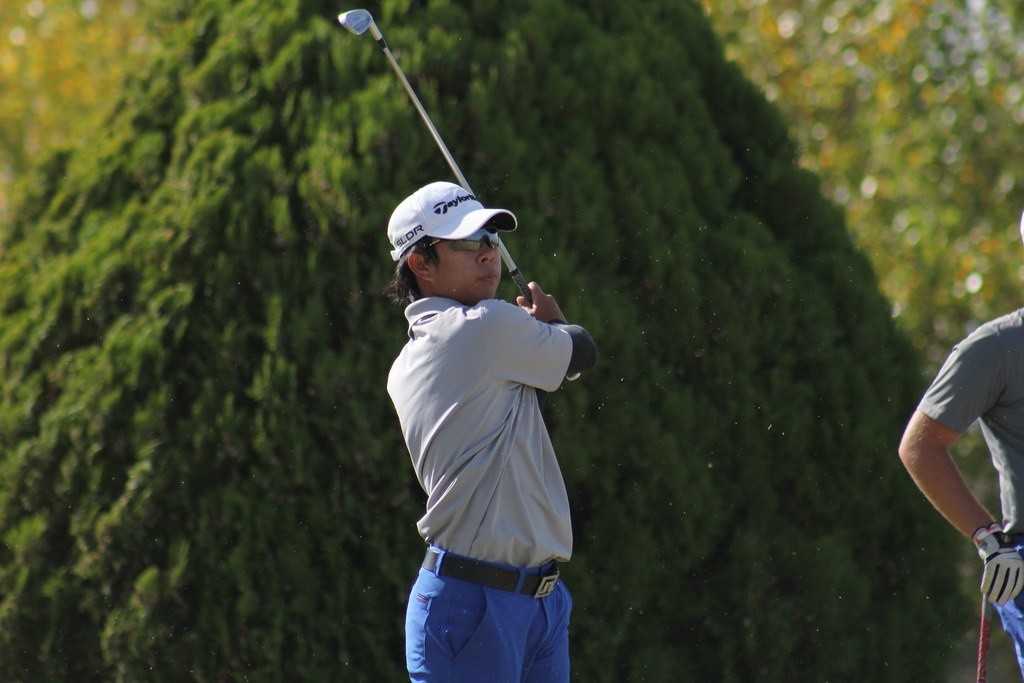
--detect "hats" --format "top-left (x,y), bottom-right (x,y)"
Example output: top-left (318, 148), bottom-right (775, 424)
top-left (387, 181), bottom-right (517, 262)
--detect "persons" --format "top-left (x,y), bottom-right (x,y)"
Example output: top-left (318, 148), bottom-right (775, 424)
top-left (386, 181), bottom-right (598, 683)
top-left (898, 308), bottom-right (1024, 678)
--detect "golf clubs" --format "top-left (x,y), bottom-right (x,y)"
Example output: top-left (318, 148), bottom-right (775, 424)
top-left (337, 8), bottom-right (582, 382)
top-left (976, 592), bottom-right (994, 683)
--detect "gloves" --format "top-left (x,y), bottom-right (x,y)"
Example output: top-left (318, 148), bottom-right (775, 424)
top-left (972, 522), bottom-right (1024, 607)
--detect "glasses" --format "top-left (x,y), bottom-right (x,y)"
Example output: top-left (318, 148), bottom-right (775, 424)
top-left (417, 226), bottom-right (499, 254)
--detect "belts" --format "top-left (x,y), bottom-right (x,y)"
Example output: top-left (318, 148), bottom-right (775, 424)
top-left (422, 547), bottom-right (560, 599)
